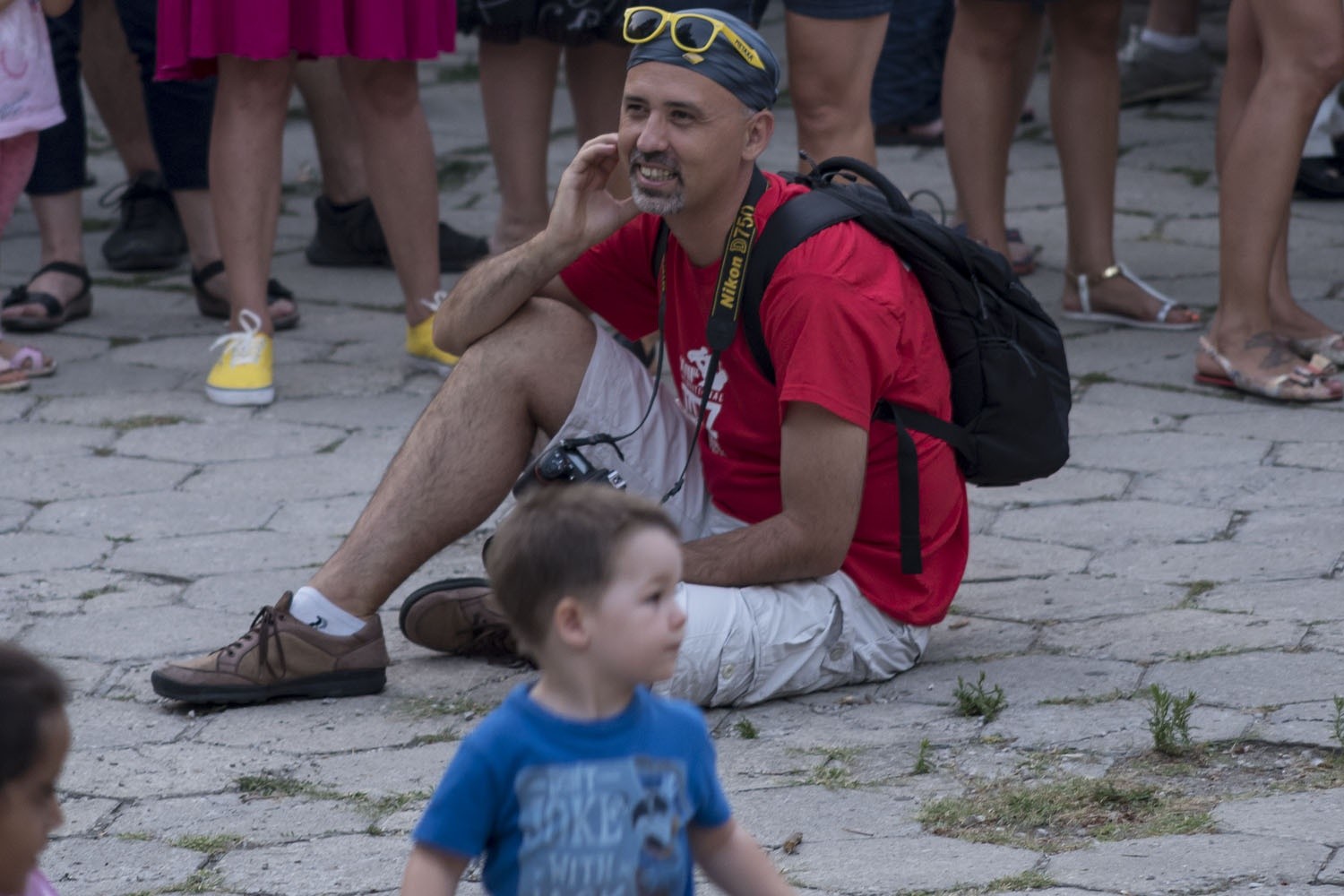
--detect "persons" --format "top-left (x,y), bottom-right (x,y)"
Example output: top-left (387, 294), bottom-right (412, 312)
top-left (0, 0), bottom-right (1344, 407)
top-left (0, 639), bottom-right (74, 896)
top-left (399, 484), bottom-right (798, 896)
top-left (941, 0), bottom-right (1204, 332)
top-left (145, 6), bottom-right (971, 708)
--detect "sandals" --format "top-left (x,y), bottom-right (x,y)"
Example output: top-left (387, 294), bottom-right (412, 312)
top-left (0, 260), bottom-right (94, 331)
top-left (0, 345), bottom-right (56, 390)
top-left (192, 260), bottom-right (300, 330)
top-left (1195, 327), bottom-right (1343, 402)
top-left (1062, 262), bottom-right (1202, 331)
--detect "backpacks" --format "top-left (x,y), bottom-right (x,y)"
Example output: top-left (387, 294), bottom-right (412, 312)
top-left (649, 157), bottom-right (1074, 488)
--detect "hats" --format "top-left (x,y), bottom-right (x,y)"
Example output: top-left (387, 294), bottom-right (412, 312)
top-left (627, 10), bottom-right (779, 112)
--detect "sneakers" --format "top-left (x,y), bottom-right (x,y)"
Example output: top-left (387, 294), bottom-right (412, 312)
top-left (149, 590), bottom-right (391, 701)
top-left (395, 578), bottom-right (536, 672)
top-left (306, 193), bottom-right (489, 273)
top-left (205, 309), bottom-right (270, 406)
top-left (405, 291), bottom-right (459, 381)
top-left (98, 169), bottom-right (187, 271)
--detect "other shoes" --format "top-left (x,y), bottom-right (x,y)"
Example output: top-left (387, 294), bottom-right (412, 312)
top-left (873, 107), bottom-right (1040, 143)
top-left (1114, 33), bottom-right (1210, 106)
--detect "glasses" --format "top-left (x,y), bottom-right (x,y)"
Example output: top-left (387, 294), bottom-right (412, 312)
top-left (622, 6), bottom-right (764, 71)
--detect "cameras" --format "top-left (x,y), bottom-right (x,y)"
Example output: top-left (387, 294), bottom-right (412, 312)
top-left (482, 442), bottom-right (627, 572)
